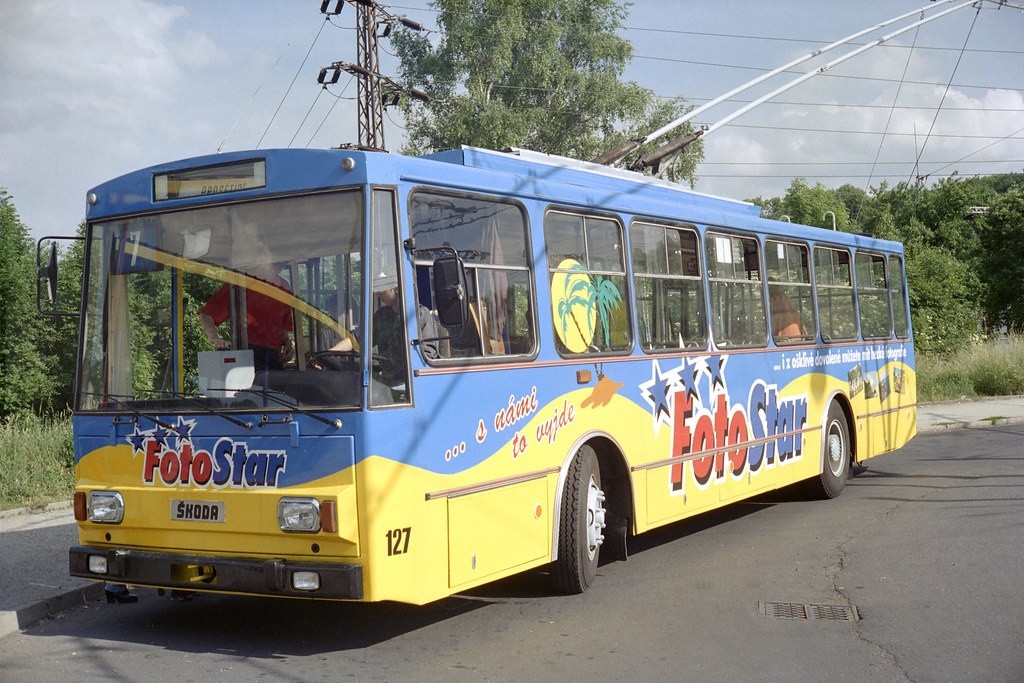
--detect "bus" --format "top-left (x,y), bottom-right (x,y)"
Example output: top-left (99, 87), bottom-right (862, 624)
top-left (38, 144), bottom-right (919, 607)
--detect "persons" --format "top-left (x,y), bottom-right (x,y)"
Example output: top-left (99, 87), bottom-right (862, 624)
top-left (199, 256), bottom-right (296, 371)
top-left (312, 265), bottom-right (439, 399)
top-left (770, 291), bottom-right (808, 343)
